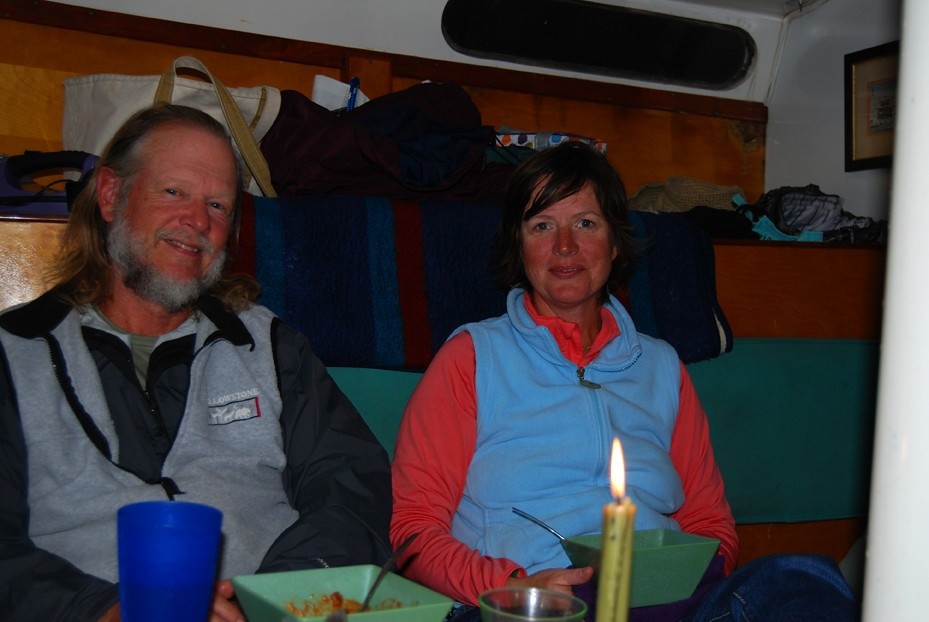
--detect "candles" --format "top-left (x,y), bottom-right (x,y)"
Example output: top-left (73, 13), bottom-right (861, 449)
top-left (595, 437), bottom-right (636, 622)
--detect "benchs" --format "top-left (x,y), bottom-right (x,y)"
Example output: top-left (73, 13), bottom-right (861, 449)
top-left (326, 337), bottom-right (879, 565)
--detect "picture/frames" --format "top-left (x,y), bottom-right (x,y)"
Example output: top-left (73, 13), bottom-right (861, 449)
top-left (844, 39), bottom-right (899, 172)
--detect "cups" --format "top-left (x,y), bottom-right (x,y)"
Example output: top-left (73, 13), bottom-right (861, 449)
top-left (477, 585), bottom-right (588, 622)
top-left (116, 500), bottom-right (224, 622)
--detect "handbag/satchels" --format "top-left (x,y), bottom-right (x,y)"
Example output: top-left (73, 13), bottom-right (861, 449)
top-left (684, 205), bottom-right (753, 239)
top-left (312, 74), bottom-right (370, 110)
top-left (732, 194), bottom-right (823, 242)
top-left (778, 192), bottom-right (845, 234)
top-left (625, 175), bottom-right (748, 211)
top-left (483, 145), bottom-right (537, 166)
top-left (259, 81), bottom-right (498, 197)
top-left (63, 56), bottom-right (281, 197)
top-left (736, 186), bottom-right (851, 244)
top-left (680, 552), bottom-right (860, 622)
top-left (495, 130), bottom-right (607, 157)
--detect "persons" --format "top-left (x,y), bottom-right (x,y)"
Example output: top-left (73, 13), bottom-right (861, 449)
top-left (0, 101), bottom-right (393, 622)
top-left (389, 138), bottom-right (861, 622)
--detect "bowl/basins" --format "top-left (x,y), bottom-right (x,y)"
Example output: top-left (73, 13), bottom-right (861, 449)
top-left (560, 527), bottom-right (722, 608)
top-left (231, 563), bottom-right (454, 622)
